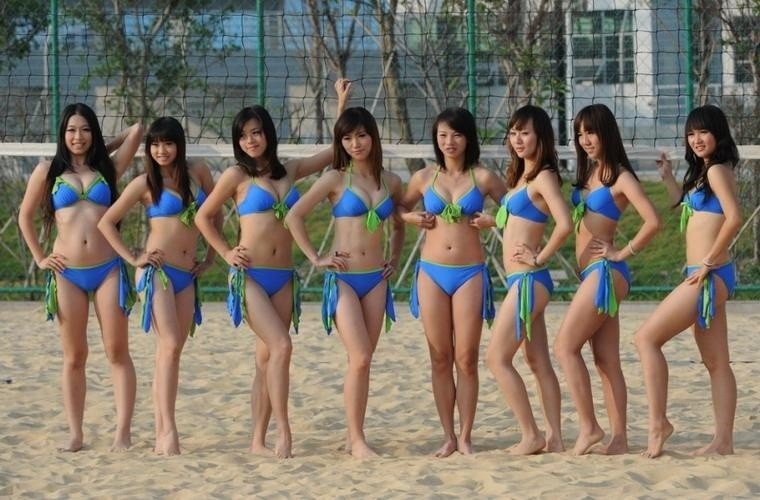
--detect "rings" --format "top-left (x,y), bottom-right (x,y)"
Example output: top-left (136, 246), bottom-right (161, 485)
top-left (335, 251), bottom-right (338, 256)
top-left (471, 219), bottom-right (474, 223)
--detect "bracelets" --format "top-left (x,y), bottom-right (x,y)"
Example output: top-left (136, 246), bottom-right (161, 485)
top-left (533, 256), bottom-right (545, 267)
top-left (702, 258), bottom-right (716, 267)
top-left (628, 240), bottom-right (639, 257)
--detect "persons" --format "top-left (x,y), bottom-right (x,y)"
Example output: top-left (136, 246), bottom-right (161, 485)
top-left (192, 76), bottom-right (352, 459)
top-left (284, 105), bottom-right (406, 461)
top-left (485, 106), bottom-right (574, 460)
top-left (17, 104), bottom-right (143, 454)
top-left (94, 117), bottom-right (224, 459)
top-left (549, 104), bottom-right (662, 458)
top-left (633, 104), bottom-right (744, 460)
top-left (393, 106), bottom-right (509, 460)
